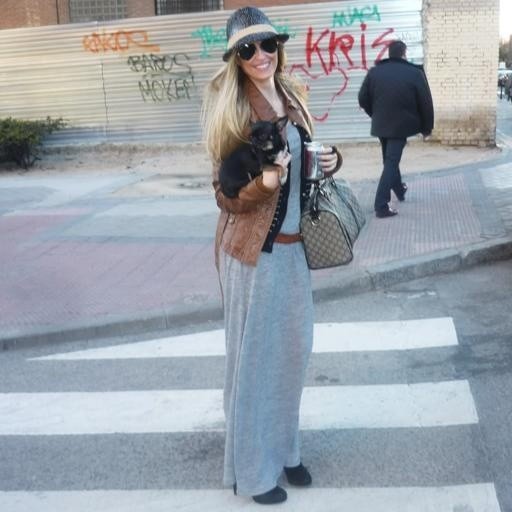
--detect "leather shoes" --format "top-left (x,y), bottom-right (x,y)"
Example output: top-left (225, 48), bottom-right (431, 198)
top-left (376, 208), bottom-right (399, 218)
top-left (233, 480), bottom-right (288, 505)
top-left (400, 182), bottom-right (408, 201)
top-left (283, 462), bottom-right (312, 486)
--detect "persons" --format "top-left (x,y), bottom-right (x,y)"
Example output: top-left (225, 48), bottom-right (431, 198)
top-left (212, 25), bottom-right (338, 504)
top-left (358, 41), bottom-right (434, 217)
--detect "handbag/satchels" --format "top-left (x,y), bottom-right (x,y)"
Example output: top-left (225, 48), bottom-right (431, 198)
top-left (298, 174), bottom-right (366, 270)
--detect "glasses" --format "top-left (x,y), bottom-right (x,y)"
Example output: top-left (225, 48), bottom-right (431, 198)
top-left (238, 38), bottom-right (279, 61)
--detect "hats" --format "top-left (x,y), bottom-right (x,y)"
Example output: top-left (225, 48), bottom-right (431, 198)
top-left (222, 6), bottom-right (289, 63)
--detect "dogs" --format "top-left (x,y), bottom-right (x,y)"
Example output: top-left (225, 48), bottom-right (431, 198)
top-left (219, 101), bottom-right (290, 200)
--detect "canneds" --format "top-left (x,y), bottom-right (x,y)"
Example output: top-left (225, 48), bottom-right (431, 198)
top-left (303, 142), bottom-right (324, 181)
